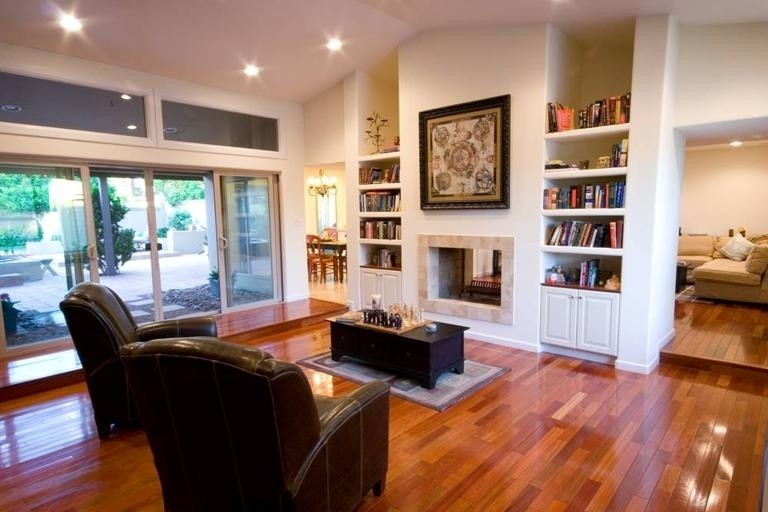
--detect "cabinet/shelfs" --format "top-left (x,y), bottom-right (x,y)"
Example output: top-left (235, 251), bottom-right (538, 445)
top-left (359, 265), bottom-right (402, 313)
top-left (543, 122), bottom-right (636, 293)
top-left (345, 150), bottom-right (402, 275)
top-left (541, 287), bottom-right (619, 366)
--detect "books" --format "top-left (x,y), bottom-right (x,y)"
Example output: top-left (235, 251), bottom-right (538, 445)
top-left (580, 258), bottom-right (600, 287)
top-left (547, 92), bottom-right (629, 133)
top-left (611, 139), bottom-right (627, 167)
top-left (543, 180), bottom-right (625, 210)
top-left (359, 165), bottom-right (400, 184)
top-left (360, 191), bottom-right (401, 212)
top-left (549, 220), bottom-right (622, 248)
top-left (360, 219), bottom-right (401, 240)
top-left (378, 248), bottom-right (397, 267)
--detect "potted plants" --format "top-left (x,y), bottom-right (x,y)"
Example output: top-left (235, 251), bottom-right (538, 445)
top-left (0, 292), bottom-right (24, 334)
top-left (208, 264), bottom-right (239, 300)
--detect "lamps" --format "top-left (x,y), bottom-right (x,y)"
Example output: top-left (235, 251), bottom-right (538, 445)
top-left (307, 168), bottom-right (338, 199)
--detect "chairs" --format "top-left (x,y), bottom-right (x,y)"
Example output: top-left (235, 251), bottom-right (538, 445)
top-left (306, 227), bottom-right (346, 284)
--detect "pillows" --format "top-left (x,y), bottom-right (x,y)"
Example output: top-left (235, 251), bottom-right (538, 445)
top-left (713, 236), bottom-right (731, 258)
top-left (720, 233), bottom-right (755, 262)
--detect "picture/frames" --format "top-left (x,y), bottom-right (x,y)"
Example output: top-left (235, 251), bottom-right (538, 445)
top-left (418, 94), bottom-right (510, 210)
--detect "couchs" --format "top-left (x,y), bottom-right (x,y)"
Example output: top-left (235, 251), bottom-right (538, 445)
top-left (677, 233), bottom-right (768, 304)
top-left (119, 337), bottom-right (390, 511)
top-left (59, 282), bottom-right (217, 440)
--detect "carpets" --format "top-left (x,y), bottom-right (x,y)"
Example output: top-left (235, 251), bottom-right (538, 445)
top-left (675, 284), bottom-right (714, 305)
top-left (296, 350), bottom-right (511, 413)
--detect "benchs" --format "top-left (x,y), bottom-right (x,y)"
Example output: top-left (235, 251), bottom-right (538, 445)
top-left (27, 255), bottom-right (58, 276)
